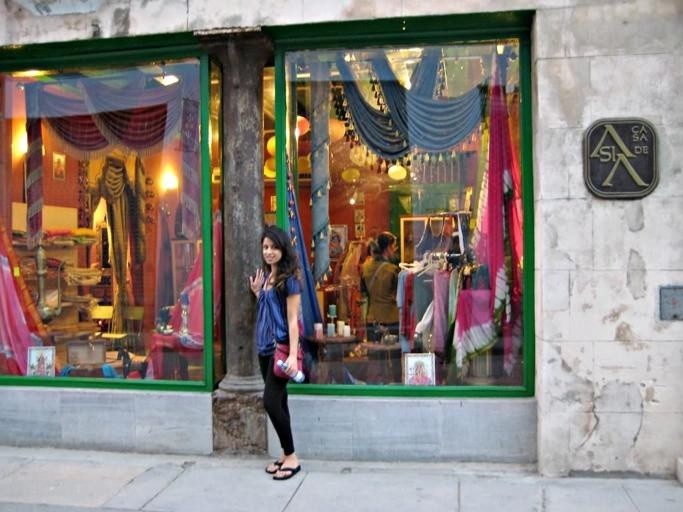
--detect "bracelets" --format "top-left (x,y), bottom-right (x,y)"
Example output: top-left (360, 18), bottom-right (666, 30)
top-left (253, 288), bottom-right (260, 293)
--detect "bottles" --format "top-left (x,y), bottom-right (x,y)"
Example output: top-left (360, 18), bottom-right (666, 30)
top-left (276, 359), bottom-right (305, 383)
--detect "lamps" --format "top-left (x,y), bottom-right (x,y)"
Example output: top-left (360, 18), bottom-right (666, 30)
top-left (263, 100), bottom-right (411, 188)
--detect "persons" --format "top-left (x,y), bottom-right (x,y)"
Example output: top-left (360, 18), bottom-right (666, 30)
top-left (54, 157), bottom-right (64, 178)
top-left (245, 225), bottom-right (303, 480)
top-left (360, 229), bottom-right (406, 385)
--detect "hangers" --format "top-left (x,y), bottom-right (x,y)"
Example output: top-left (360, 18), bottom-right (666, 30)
top-left (395, 249), bottom-right (467, 282)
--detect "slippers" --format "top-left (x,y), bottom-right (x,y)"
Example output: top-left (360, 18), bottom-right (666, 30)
top-left (264, 459), bottom-right (301, 479)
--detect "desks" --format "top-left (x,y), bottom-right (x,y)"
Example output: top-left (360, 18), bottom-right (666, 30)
top-left (306, 331), bottom-right (402, 390)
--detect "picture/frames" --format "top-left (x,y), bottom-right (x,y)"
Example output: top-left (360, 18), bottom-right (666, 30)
top-left (52, 152), bottom-right (66, 181)
top-left (327, 224), bottom-right (347, 262)
top-left (399, 351), bottom-right (437, 386)
top-left (25, 340), bottom-right (107, 378)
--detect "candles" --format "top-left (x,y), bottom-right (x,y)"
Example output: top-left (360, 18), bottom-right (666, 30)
top-left (312, 305), bottom-right (352, 339)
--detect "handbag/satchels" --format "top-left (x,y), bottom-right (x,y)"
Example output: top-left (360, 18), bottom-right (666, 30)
top-left (272, 342), bottom-right (304, 379)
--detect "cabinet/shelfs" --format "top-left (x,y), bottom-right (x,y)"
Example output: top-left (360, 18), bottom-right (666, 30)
top-left (12, 234), bottom-right (102, 317)
